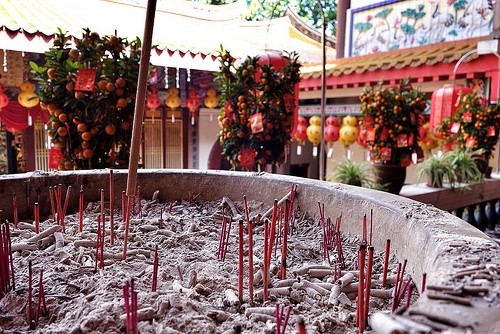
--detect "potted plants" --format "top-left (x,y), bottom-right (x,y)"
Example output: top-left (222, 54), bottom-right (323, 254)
top-left (358, 79), bottom-right (427, 194)
top-left (437, 92), bottom-right (500, 176)
top-left (419, 151), bottom-right (459, 189)
top-left (324, 160), bottom-right (386, 192)
top-left (453, 149), bottom-right (479, 182)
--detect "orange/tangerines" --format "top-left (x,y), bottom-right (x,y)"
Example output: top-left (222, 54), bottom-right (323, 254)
top-left (438, 95), bottom-right (500, 149)
top-left (217, 54), bottom-right (290, 165)
top-left (360, 87), bottom-right (426, 150)
top-left (41, 33), bottom-right (135, 171)
